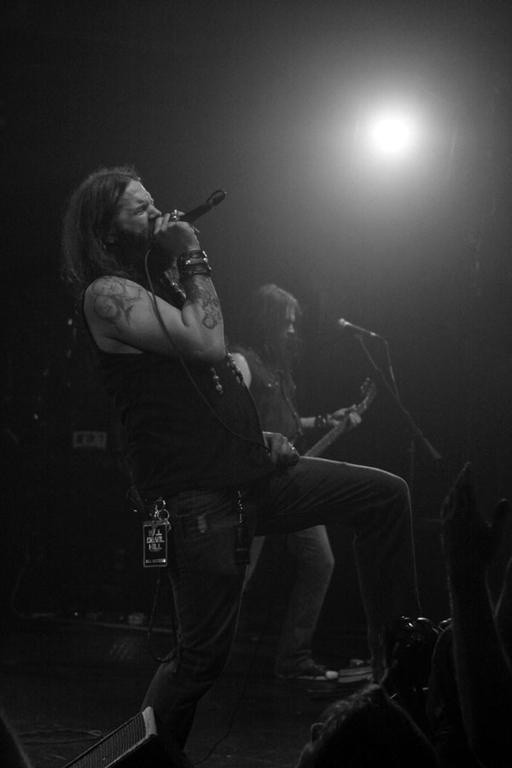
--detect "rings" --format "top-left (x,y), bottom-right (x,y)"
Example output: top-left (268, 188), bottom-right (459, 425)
top-left (170, 209), bottom-right (178, 221)
top-left (288, 441), bottom-right (296, 452)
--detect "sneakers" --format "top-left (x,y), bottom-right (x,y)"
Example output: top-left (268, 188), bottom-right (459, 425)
top-left (279, 664), bottom-right (338, 681)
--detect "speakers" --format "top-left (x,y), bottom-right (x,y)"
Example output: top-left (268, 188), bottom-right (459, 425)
top-left (61, 706), bottom-right (194, 768)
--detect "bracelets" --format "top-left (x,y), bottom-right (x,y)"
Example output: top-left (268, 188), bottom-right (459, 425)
top-left (177, 249), bottom-right (207, 266)
top-left (180, 262), bottom-right (212, 276)
top-left (313, 411), bottom-right (329, 431)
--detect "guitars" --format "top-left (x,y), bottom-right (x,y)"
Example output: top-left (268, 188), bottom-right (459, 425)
top-left (291, 377), bottom-right (377, 459)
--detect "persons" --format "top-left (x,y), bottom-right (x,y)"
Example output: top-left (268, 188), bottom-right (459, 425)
top-left (226, 284), bottom-right (359, 681)
top-left (296, 685), bottom-right (442, 767)
top-left (57, 164), bottom-right (422, 768)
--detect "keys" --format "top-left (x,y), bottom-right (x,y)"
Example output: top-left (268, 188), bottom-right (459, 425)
top-left (151, 520), bottom-right (158, 536)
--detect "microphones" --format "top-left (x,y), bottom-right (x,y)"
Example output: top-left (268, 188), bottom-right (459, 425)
top-left (334, 318), bottom-right (384, 342)
top-left (179, 189), bottom-right (226, 226)
